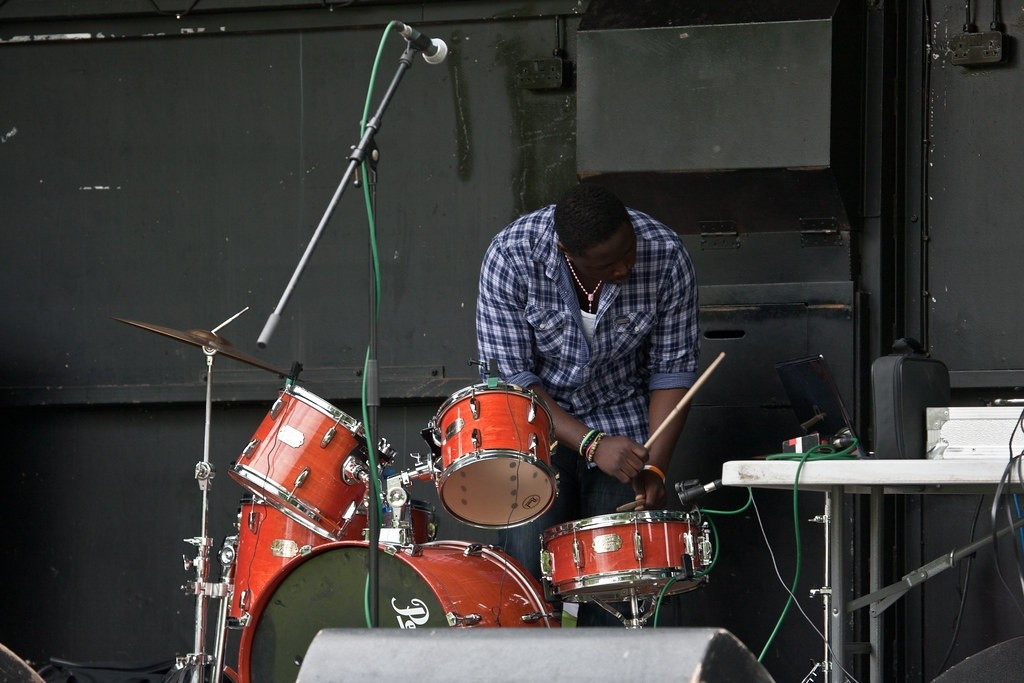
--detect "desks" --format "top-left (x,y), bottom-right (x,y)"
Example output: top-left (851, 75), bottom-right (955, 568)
top-left (723, 460), bottom-right (1024, 683)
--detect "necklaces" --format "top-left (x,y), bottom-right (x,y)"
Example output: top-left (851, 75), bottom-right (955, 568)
top-left (564, 252), bottom-right (605, 316)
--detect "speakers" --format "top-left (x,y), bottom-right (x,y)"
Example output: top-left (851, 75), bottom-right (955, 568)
top-left (0, 643), bottom-right (45, 683)
top-left (295, 627), bottom-right (777, 683)
top-left (930, 635), bottom-right (1024, 683)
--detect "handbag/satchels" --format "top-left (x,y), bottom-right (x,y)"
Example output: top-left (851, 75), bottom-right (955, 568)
top-left (872, 336), bottom-right (951, 459)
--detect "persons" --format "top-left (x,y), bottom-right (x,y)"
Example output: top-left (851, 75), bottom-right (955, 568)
top-left (472, 182), bottom-right (702, 629)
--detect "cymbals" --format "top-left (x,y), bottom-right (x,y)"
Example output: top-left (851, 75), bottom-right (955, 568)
top-left (110, 314), bottom-right (306, 382)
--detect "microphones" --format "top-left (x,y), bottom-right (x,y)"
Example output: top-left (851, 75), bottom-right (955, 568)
top-left (391, 21), bottom-right (448, 64)
top-left (677, 479), bottom-right (723, 506)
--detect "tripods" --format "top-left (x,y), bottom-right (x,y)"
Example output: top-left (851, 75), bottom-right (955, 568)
top-left (160, 347), bottom-right (239, 683)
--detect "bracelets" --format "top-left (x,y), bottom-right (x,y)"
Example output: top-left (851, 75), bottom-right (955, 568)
top-left (579, 427), bottom-right (608, 469)
top-left (643, 463), bottom-right (666, 485)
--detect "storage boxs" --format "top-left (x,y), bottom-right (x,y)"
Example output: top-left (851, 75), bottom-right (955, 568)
top-left (927, 406), bottom-right (1024, 460)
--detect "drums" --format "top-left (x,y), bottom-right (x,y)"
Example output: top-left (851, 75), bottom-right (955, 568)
top-left (422, 374), bottom-right (560, 531)
top-left (231, 493), bottom-right (437, 626)
top-left (535, 504), bottom-right (712, 601)
top-left (236, 534), bottom-right (562, 683)
top-left (226, 373), bottom-right (396, 542)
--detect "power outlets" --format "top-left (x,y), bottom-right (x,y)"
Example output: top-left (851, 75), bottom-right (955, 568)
top-left (950, 31), bottom-right (1011, 68)
top-left (514, 57), bottom-right (573, 89)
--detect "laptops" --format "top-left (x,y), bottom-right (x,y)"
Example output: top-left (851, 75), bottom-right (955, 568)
top-left (777, 355), bottom-right (875, 460)
